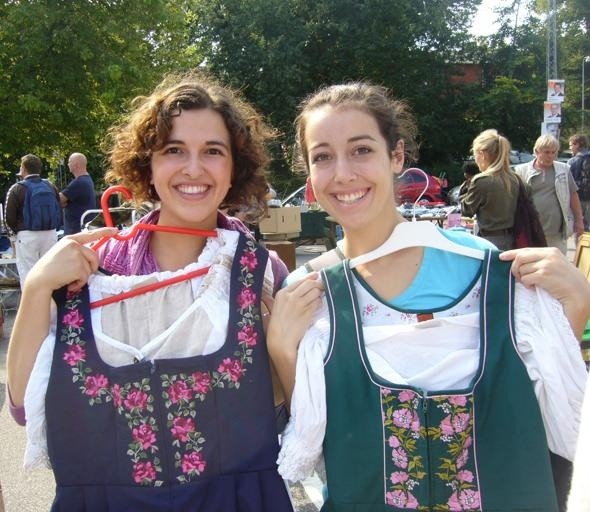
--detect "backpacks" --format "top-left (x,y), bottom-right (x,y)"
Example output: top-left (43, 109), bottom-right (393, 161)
top-left (16, 179), bottom-right (62, 230)
top-left (577, 153), bottom-right (590, 200)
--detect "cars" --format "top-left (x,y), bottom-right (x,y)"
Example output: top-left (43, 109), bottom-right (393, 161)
top-left (303, 162), bottom-right (449, 210)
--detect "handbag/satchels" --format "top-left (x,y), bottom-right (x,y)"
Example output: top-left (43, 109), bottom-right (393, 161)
top-left (512, 172), bottom-right (548, 247)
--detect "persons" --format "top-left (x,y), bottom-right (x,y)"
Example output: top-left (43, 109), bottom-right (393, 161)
top-left (3, 152), bottom-right (62, 293)
top-left (57, 151), bottom-right (99, 236)
top-left (265, 77), bottom-right (590, 508)
top-left (5, 72), bottom-right (294, 508)
top-left (456, 162), bottom-right (480, 199)
top-left (515, 133), bottom-right (585, 257)
top-left (567, 133), bottom-right (590, 240)
top-left (461, 128), bottom-right (524, 250)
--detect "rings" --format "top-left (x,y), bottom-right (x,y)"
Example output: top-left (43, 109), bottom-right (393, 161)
top-left (261, 311), bottom-right (270, 317)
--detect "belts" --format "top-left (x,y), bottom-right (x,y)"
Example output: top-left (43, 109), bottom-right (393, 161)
top-left (477, 228), bottom-right (513, 236)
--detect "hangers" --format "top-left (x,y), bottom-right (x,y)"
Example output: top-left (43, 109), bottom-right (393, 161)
top-left (86, 185), bottom-right (217, 308)
top-left (347, 168), bottom-right (486, 269)
